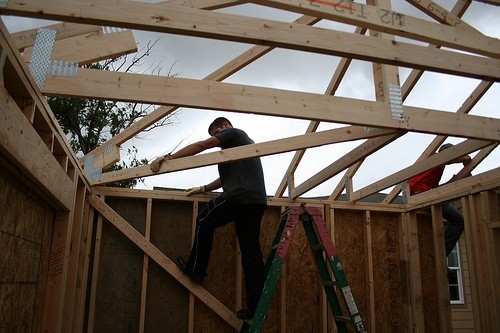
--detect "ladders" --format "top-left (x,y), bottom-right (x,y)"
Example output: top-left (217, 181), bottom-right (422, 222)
top-left (249, 202), bottom-right (364, 333)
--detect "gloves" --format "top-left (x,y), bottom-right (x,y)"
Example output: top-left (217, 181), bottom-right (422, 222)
top-left (185, 186), bottom-right (207, 196)
top-left (150, 153), bottom-right (173, 173)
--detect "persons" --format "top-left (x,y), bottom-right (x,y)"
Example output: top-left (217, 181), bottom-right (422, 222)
top-left (408, 144), bottom-right (472, 280)
top-left (150, 117), bottom-right (267, 317)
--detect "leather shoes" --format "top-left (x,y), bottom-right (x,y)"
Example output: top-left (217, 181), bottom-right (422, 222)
top-left (177, 256), bottom-right (201, 284)
top-left (237, 310), bottom-right (267, 320)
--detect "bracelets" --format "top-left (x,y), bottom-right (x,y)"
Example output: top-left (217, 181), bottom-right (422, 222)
top-left (204, 185), bottom-right (207, 192)
